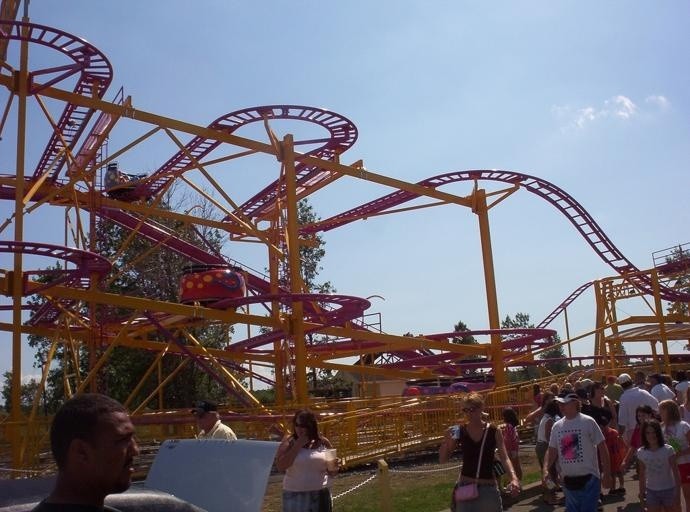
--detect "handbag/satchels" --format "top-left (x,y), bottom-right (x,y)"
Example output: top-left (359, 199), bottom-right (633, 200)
top-left (454, 484), bottom-right (478, 500)
top-left (563, 477), bottom-right (586, 490)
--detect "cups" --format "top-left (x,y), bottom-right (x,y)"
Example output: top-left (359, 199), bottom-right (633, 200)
top-left (325, 449), bottom-right (339, 472)
top-left (449, 426), bottom-right (460, 439)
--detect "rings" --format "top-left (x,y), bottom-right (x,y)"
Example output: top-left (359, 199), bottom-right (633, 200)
top-left (518, 490), bottom-right (521, 493)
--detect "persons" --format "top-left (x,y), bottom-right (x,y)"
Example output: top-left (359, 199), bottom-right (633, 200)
top-left (191, 400), bottom-right (237, 440)
top-left (31, 393), bottom-right (139, 512)
top-left (498, 406), bottom-right (522, 493)
top-left (523, 370), bottom-right (690, 512)
top-left (438, 391), bottom-right (521, 512)
top-left (275, 409), bottom-right (342, 512)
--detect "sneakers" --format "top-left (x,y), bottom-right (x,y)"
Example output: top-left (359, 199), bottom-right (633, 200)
top-left (610, 487), bottom-right (626, 496)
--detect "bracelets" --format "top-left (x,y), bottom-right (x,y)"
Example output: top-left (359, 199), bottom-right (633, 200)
top-left (509, 471), bottom-right (516, 474)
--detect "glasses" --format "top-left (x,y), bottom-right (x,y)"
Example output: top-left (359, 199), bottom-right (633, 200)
top-left (462, 405), bottom-right (481, 414)
top-left (293, 421), bottom-right (316, 429)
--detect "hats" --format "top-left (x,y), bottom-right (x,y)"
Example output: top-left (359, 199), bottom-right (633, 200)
top-left (617, 373), bottom-right (633, 383)
top-left (193, 397), bottom-right (221, 416)
top-left (555, 393), bottom-right (583, 403)
